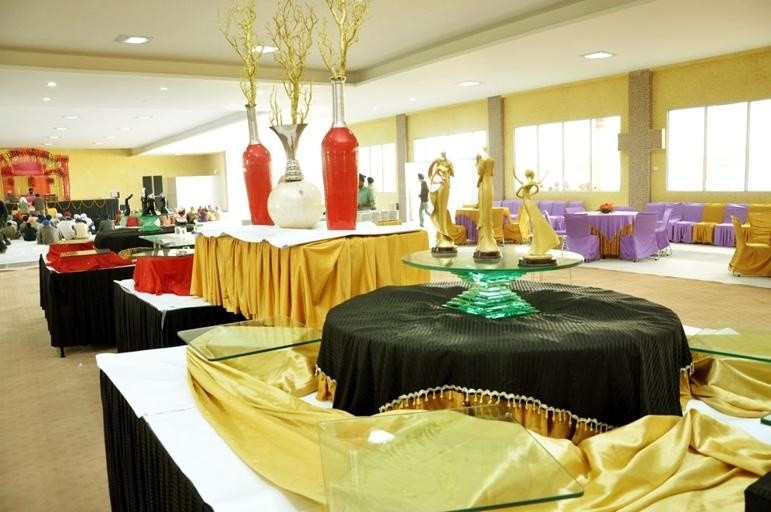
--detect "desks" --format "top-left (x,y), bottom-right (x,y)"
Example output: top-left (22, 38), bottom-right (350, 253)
top-left (48, 198), bottom-right (119, 220)
top-left (39, 208), bottom-right (771, 512)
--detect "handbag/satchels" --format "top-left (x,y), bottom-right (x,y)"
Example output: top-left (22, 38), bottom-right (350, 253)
top-left (677, 357), bottom-right (769, 420)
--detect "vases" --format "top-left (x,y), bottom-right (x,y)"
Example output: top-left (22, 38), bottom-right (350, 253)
top-left (243, 104), bottom-right (274, 226)
top-left (267, 123), bottom-right (323, 230)
top-left (323, 75), bottom-right (360, 229)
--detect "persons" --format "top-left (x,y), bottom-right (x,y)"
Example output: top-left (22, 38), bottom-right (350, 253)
top-left (357, 172), bottom-right (376, 212)
top-left (415, 172), bottom-right (431, 227)
top-left (366, 176), bottom-right (376, 210)
top-left (473, 154), bottom-right (501, 259)
top-left (0, 187), bottom-right (222, 255)
top-left (513, 169), bottom-right (561, 256)
top-left (422, 151), bottom-right (456, 248)
